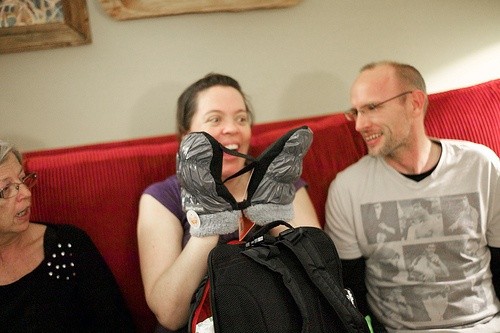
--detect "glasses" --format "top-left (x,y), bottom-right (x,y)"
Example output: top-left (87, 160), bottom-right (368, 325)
top-left (343, 91), bottom-right (413, 121)
top-left (0, 172), bottom-right (38, 199)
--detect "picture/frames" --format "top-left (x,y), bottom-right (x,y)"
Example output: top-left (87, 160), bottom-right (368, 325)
top-left (0, 0), bottom-right (93, 54)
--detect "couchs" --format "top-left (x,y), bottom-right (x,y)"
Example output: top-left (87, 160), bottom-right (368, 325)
top-left (19, 78), bottom-right (500, 332)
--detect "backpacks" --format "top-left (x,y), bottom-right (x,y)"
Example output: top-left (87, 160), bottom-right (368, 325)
top-left (188, 220), bottom-right (371, 333)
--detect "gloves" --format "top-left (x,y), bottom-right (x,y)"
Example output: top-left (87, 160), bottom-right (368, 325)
top-left (176, 131), bottom-right (243, 237)
top-left (242, 125), bottom-right (314, 227)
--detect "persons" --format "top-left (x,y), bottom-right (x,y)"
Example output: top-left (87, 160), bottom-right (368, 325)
top-left (324, 61), bottom-right (500, 333)
top-left (0, 139), bottom-right (141, 333)
top-left (136, 71), bottom-right (337, 332)
top-left (360, 188), bottom-right (499, 330)
